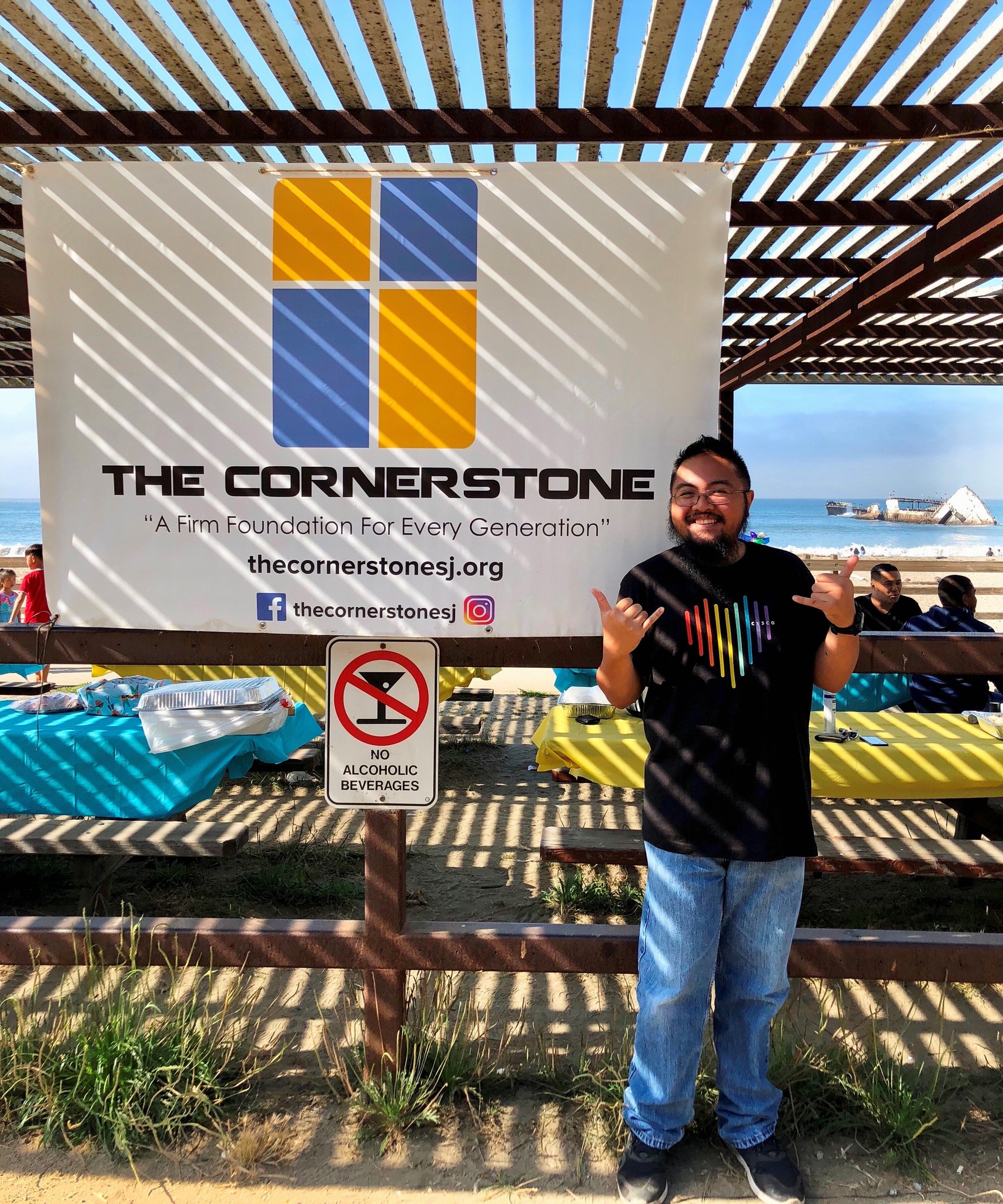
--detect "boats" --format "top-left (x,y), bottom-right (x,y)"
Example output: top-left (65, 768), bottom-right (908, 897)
top-left (825, 500), bottom-right (868, 516)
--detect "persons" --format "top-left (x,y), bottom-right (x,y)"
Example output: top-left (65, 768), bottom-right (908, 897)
top-left (897, 574), bottom-right (1003, 713)
top-left (8, 544), bottom-right (53, 684)
top-left (0, 568), bottom-right (21, 623)
top-left (853, 563), bottom-right (925, 631)
top-left (986, 548), bottom-right (1003, 557)
top-left (589, 436), bottom-right (864, 1204)
top-left (844, 546), bottom-right (866, 558)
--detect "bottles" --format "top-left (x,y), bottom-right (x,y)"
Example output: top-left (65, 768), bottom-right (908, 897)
top-left (823, 690), bottom-right (836, 736)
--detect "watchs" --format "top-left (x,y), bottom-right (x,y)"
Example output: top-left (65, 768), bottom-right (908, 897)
top-left (829, 599), bottom-right (864, 636)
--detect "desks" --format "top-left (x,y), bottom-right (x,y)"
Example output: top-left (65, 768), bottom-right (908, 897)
top-left (541, 701), bottom-right (1003, 801)
top-left (0, 697), bottom-right (305, 823)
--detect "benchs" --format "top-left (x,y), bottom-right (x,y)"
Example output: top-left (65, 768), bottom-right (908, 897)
top-left (537, 825), bottom-right (1003, 879)
top-left (854, 631), bottom-right (1003, 681)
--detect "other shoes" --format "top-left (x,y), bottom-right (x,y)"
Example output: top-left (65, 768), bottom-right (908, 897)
top-left (551, 770), bottom-right (595, 783)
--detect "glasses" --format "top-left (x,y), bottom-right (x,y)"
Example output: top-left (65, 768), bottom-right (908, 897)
top-left (671, 487), bottom-right (750, 507)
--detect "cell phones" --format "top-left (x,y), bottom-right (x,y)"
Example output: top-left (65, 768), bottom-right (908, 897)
top-left (859, 736), bottom-right (889, 746)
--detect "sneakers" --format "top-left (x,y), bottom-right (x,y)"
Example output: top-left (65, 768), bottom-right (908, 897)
top-left (616, 1130), bottom-right (668, 1204)
top-left (727, 1134), bottom-right (805, 1204)
